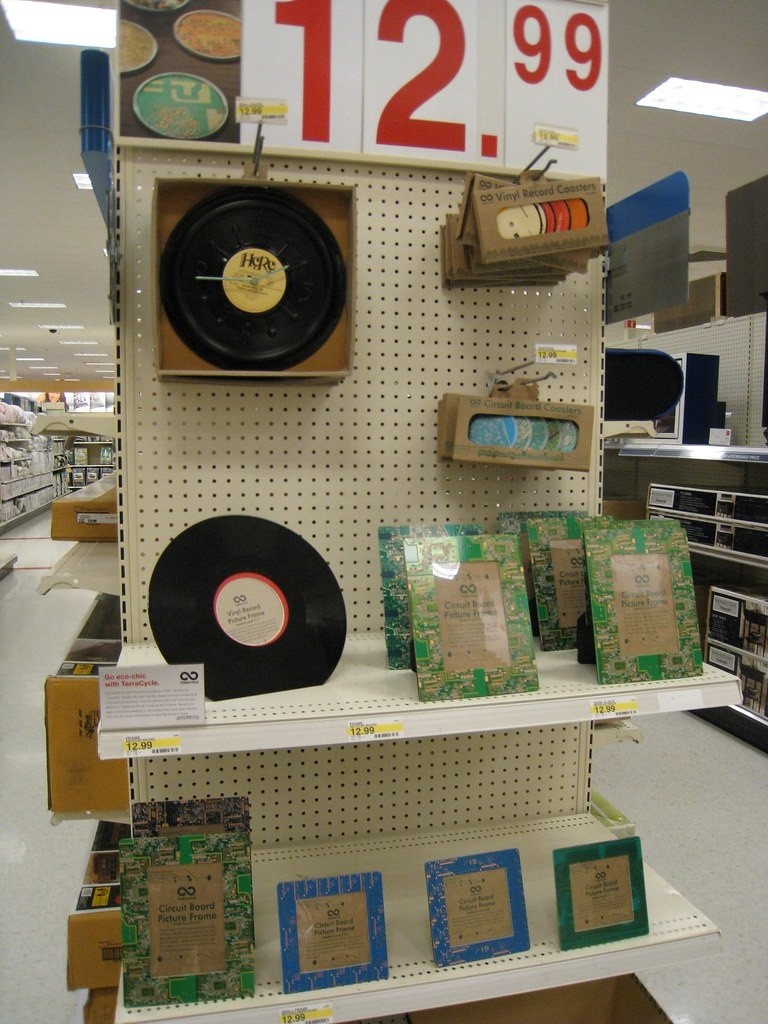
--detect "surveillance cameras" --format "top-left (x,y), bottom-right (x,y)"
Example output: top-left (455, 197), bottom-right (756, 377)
top-left (49, 329), bottom-right (57, 333)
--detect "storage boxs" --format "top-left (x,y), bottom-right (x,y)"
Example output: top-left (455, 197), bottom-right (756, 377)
top-left (646, 482), bottom-right (768, 561)
top-left (605, 349), bottom-right (733, 446)
top-left (83, 986), bottom-right (119, 1024)
top-left (66, 820), bottom-right (131, 992)
top-left (705, 581), bottom-right (768, 721)
top-left (601, 496), bottom-right (646, 520)
top-left (334, 972), bottom-right (674, 1024)
top-left (50, 470), bottom-right (117, 542)
top-left (43, 594), bottom-right (129, 813)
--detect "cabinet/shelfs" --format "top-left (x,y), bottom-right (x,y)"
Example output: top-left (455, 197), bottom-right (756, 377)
top-left (29, 409), bottom-right (133, 827)
top-left (96, 629), bottom-right (744, 1024)
top-left (617, 438), bottom-right (768, 756)
top-left (0, 422), bottom-right (73, 537)
top-left (67, 441), bottom-right (115, 489)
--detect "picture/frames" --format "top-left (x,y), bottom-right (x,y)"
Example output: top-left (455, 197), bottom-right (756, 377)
top-left (117, 506), bottom-right (713, 1008)
